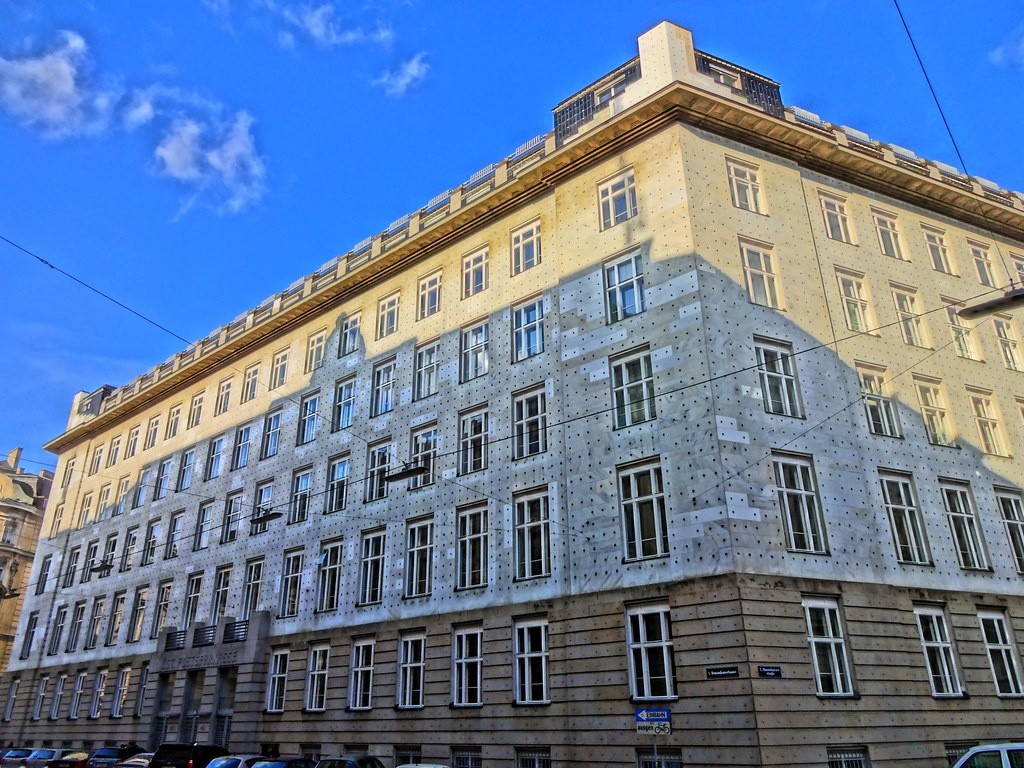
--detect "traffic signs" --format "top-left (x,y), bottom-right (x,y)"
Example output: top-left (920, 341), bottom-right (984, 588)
top-left (635, 708), bottom-right (672, 736)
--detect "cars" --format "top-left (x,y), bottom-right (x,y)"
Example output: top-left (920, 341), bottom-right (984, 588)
top-left (250, 756), bottom-right (317, 768)
top-left (47, 750), bottom-right (96, 768)
top-left (394, 763), bottom-right (449, 768)
top-left (205, 753), bottom-right (270, 768)
top-left (114, 751), bottom-right (156, 768)
top-left (949, 742), bottom-right (1024, 768)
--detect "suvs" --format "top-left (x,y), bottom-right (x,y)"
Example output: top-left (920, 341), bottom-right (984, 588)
top-left (145, 740), bottom-right (231, 768)
top-left (313, 753), bottom-right (386, 768)
top-left (85, 744), bottom-right (147, 768)
top-left (0, 747), bottom-right (77, 768)
